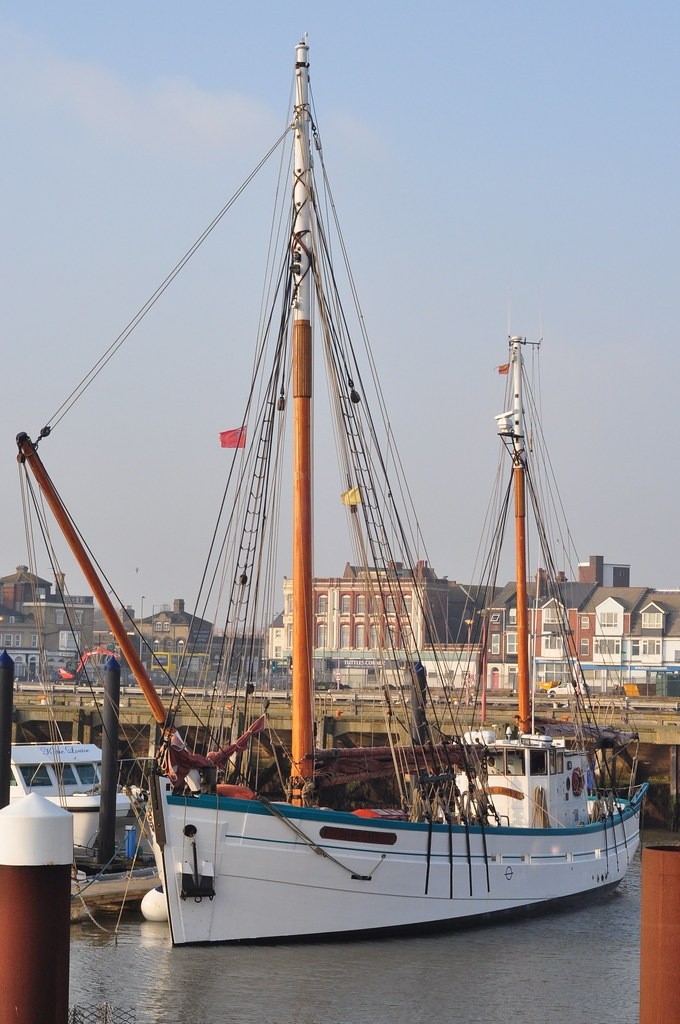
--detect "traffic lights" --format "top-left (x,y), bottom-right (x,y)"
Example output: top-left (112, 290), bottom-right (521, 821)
top-left (272, 661), bottom-right (276, 671)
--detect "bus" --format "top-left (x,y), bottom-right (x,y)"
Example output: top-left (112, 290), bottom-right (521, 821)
top-left (151, 652), bottom-right (211, 671)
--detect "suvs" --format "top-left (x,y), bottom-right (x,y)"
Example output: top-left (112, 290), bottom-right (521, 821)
top-left (547, 682), bottom-right (591, 699)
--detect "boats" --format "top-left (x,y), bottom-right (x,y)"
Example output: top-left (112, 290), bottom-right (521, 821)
top-left (10, 740), bottom-right (162, 872)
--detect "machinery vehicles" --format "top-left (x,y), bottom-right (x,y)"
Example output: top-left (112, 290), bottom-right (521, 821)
top-left (59, 647), bottom-right (124, 687)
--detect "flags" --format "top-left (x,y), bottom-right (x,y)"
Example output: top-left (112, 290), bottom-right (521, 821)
top-left (340, 485), bottom-right (362, 504)
top-left (219, 425), bottom-right (247, 448)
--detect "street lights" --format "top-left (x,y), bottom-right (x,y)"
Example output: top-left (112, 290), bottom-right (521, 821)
top-left (464, 619), bottom-right (475, 706)
top-left (140, 596), bottom-right (146, 661)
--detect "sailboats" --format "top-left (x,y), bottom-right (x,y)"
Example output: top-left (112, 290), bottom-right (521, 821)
top-left (16, 38), bottom-right (656, 946)
